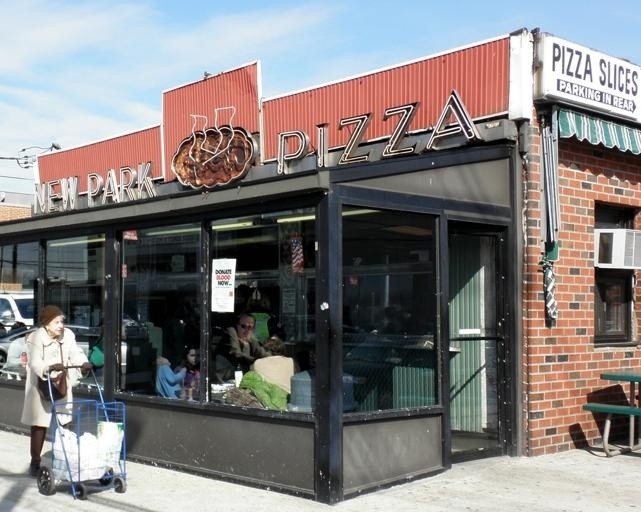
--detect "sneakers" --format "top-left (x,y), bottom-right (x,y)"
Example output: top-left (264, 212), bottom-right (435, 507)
top-left (30, 463), bottom-right (41, 475)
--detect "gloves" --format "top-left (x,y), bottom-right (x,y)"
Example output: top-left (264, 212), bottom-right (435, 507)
top-left (82, 363), bottom-right (93, 370)
top-left (47, 363), bottom-right (65, 372)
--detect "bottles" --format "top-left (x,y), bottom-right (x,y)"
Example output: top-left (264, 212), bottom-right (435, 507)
top-left (234, 361), bottom-right (243, 386)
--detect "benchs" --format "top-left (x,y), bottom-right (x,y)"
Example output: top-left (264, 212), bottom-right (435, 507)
top-left (581, 401), bottom-right (640, 456)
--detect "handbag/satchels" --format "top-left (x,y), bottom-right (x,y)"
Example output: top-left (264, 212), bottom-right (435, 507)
top-left (37, 370), bottom-right (67, 402)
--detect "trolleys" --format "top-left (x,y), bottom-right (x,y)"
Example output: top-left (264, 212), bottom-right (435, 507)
top-left (37, 365), bottom-right (127, 500)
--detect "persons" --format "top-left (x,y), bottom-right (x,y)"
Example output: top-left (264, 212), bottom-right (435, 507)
top-left (19, 303), bottom-right (93, 478)
top-left (212, 311), bottom-right (267, 385)
top-left (250, 334), bottom-right (303, 407)
top-left (152, 341), bottom-right (200, 404)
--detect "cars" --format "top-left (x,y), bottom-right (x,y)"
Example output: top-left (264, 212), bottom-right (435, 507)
top-left (0, 288), bottom-right (129, 368)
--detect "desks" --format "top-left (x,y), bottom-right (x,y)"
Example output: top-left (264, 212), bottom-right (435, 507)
top-left (599, 370), bottom-right (641, 452)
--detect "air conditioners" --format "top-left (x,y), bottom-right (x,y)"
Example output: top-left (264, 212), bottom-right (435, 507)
top-left (593, 228), bottom-right (641, 270)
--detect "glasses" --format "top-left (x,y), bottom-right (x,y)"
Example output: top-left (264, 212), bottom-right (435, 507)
top-left (240, 323), bottom-right (255, 330)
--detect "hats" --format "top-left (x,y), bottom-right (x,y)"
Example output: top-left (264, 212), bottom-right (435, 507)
top-left (40, 305), bottom-right (64, 325)
